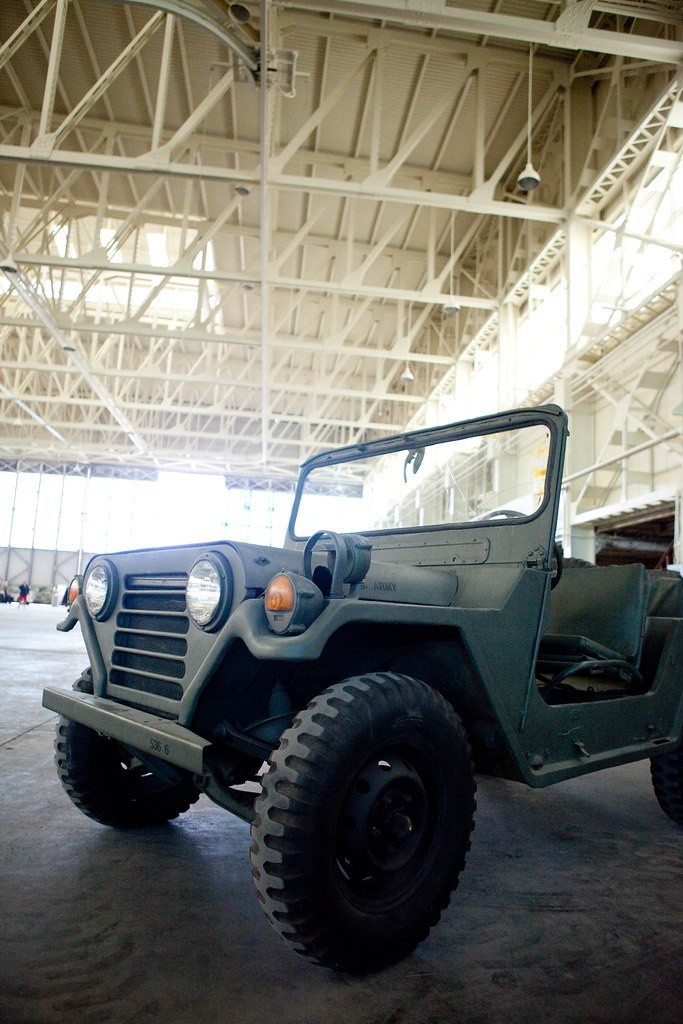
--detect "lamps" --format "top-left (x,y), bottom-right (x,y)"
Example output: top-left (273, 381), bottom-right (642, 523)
top-left (235, 183), bottom-right (250, 197)
top-left (401, 307), bottom-right (415, 381)
top-left (440, 209), bottom-right (459, 313)
top-left (241, 282), bottom-right (252, 288)
top-left (0, 159), bottom-right (26, 274)
top-left (517, 43), bottom-right (540, 190)
top-left (63, 335), bottom-right (75, 352)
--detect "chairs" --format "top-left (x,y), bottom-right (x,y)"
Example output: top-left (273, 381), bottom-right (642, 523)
top-left (533, 562), bottom-right (650, 692)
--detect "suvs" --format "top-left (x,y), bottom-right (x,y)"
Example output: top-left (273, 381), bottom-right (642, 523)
top-left (38, 405), bottom-right (683, 980)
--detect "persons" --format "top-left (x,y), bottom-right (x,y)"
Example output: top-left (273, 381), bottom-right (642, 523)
top-left (17, 581), bottom-right (29, 604)
